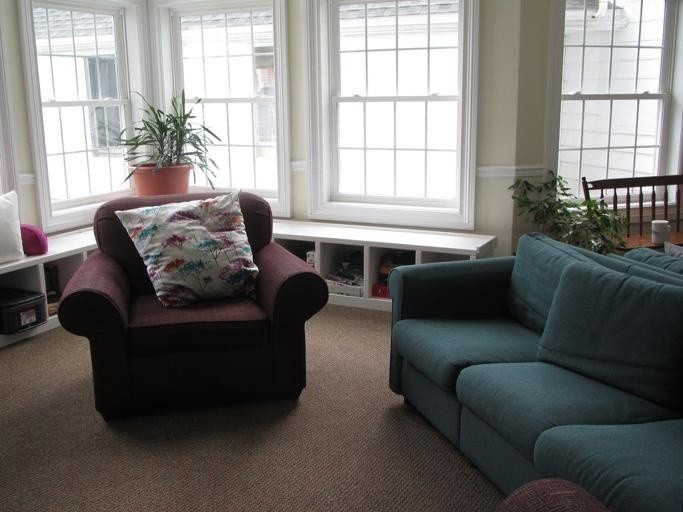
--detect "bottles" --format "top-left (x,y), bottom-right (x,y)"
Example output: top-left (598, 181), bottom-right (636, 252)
top-left (651, 220), bottom-right (668, 244)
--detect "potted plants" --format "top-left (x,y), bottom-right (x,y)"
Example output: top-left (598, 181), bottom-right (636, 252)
top-left (114, 88), bottom-right (222, 196)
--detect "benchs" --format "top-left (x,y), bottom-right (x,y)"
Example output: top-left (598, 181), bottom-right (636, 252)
top-left (581, 175), bottom-right (683, 249)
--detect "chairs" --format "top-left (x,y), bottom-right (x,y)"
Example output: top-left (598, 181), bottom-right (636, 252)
top-left (57, 189), bottom-right (329, 417)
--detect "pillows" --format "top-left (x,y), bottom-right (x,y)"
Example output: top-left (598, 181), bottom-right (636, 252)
top-left (536, 261), bottom-right (683, 412)
top-left (624, 246), bottom-right (683, 276)
top-left (663, 241), bottom-right (682, 258)
top-left (0, 190), bottom-right (23, 265)
top-left (506, 231), bottom-right (597, 335)
top-left (114, 189), bottom-right (259, 308)
top-left (20, 223), bottom-right (48, 255)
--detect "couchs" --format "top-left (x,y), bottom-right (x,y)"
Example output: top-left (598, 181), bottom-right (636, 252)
top-left (387, 242), bottom-right (683, 512)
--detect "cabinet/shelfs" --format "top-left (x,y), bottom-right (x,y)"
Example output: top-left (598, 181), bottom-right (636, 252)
top-left (0, 216), bottom-right (497, 349)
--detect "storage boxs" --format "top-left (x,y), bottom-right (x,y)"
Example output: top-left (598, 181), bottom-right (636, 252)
top-left (0, 287), bottom-right (48, 335)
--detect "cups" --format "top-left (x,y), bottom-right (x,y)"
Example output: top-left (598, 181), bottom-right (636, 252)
top-left (373, 283), bottom-right (387, 296)
